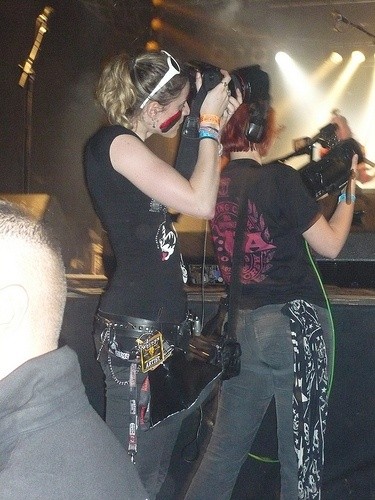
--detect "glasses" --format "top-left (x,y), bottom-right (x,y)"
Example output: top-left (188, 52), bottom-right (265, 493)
top-left (139, 50), bottom-right (181, 110)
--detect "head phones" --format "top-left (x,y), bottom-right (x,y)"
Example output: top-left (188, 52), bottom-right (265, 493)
top-left (244, 101), bottom-right (268, 143)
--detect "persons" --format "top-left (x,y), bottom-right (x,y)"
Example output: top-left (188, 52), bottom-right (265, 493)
top-left (81, 47), bottom-right (243, 499)
top-left (1, 198), bottom-right (148, 499)
top-left (186, 64), bottom-right (359, 500)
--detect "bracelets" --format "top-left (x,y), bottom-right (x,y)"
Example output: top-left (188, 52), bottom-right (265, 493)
top-left (199, 113), bottom-right (221, 126)
top-left (199, 126), bottom-right (221, 146)
top-left (337, 194), bottom-right (356, 202)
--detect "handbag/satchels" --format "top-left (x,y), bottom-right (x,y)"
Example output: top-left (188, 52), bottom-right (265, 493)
top-left (137, 351), bottom-right (226, 434)
top-left (201, 318), bottom-right (241, 381)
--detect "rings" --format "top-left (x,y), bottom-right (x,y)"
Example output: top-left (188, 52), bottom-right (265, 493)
top-left (227, 90), bottom-right (232, 97)
top-left (220, 81), bottom-right (228, 88)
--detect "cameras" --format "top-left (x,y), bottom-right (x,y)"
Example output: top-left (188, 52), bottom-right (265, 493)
top-left (184, 60), bottom-right (270, 103)
